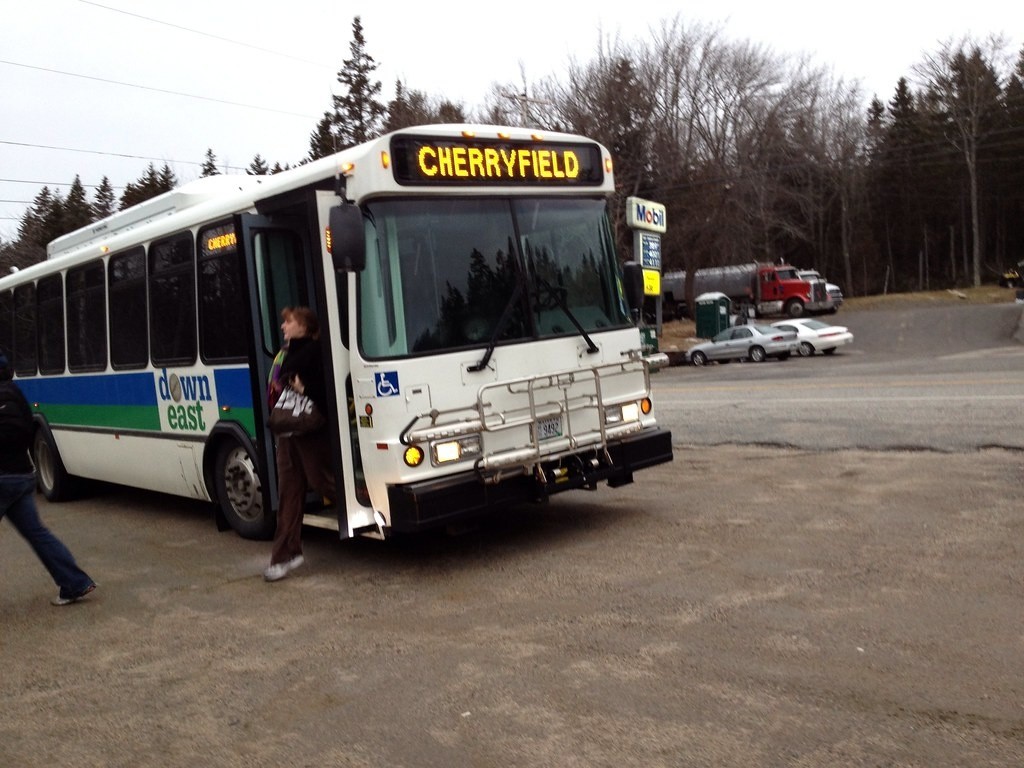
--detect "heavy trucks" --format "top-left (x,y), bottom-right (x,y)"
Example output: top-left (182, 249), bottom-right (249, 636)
top-left (661, 257), bottom-right (833, 318)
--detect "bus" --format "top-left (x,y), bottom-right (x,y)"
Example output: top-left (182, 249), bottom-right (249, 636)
top-left (0, 123), bottom-right (674, 544)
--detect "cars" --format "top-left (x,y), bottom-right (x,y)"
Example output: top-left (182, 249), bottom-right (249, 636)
top-left (769, 319), bottom-right (854, 357)
top-left (998, 260), bottom-right (1024, 288)
top-left (798, 271), bottom-right (843, 315)
top-left (683, 324), bottom-right (802, 367)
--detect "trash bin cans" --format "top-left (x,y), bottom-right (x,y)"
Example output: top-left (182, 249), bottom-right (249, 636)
top-left (695, 292), bottom-right (731, 340)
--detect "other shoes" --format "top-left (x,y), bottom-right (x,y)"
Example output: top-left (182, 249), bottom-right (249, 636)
top-left (50, 582), bottom-right (97, 606)
top-left (264, 555), bottom-right (304, 582)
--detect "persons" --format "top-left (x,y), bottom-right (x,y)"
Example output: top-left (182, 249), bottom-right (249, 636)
top-left (263, 304), bottom-right (342, 582)
top-left (0, 343), bottom-right (97, 607)
